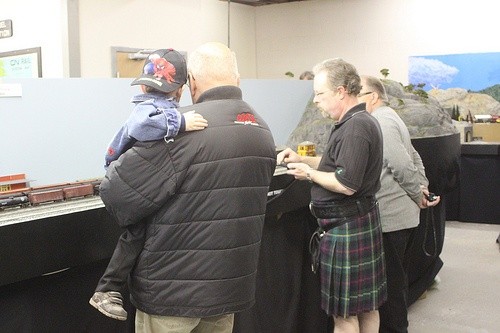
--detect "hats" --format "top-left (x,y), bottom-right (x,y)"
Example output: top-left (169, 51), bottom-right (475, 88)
top-left (130, 48), bottom-right (187, 93)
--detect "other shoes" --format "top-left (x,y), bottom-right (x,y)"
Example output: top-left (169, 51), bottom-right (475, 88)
top-left (89, 291), bottom-right (127, 321)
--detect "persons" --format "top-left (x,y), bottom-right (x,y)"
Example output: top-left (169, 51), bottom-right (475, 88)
top-left (89, 48), bottom-right (208, 319)
top-left (99, 42), bottom-right (277, 333)
top-left (358, 74), bottom-right (440, 333)
top-left (276, 58), bottom-right (388, 333)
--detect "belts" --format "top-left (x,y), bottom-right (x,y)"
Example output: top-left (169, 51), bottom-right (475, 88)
top-left (309, 194), bottom-right (378, 219)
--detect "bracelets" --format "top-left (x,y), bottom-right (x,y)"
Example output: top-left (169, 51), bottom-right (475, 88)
top-left (306, 167), bottom-right (314, 184)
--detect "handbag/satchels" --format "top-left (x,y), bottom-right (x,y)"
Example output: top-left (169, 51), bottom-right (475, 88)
top-left (308, 231), bottom-right (322, 275)
